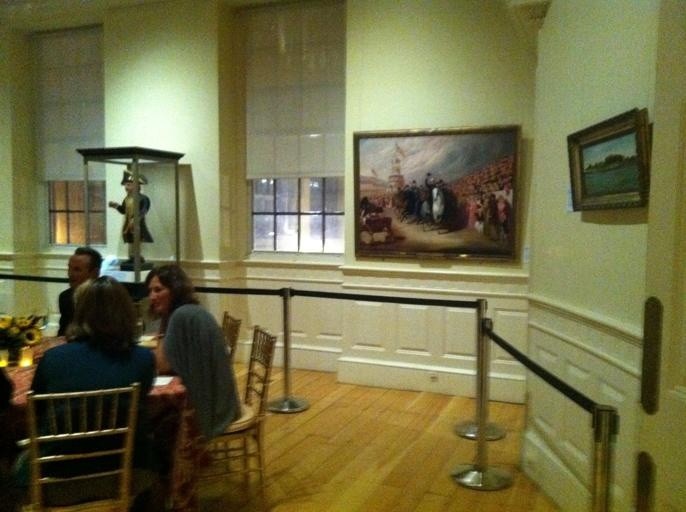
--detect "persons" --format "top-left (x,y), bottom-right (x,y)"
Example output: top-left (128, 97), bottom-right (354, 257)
top-left (108, 180), bottom-right (152, 261)
top-left (54, 246), bottom-right (102, 335)
top-left (394, 172), bottom-right (512, 241)
top-left (13, 276), bottom-right (156, 506)
top-left (144, 262), bottom-right (238, 436)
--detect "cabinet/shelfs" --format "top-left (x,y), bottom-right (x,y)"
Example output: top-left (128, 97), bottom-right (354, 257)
top-left (75, 145), bottom-right (184, 302)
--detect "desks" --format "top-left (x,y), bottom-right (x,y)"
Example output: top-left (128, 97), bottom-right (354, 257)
top-left (0, 332), bottom-right (205, 512)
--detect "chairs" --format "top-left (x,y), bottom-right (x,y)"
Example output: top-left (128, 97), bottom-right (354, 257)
top-left (8, 378), bottom-right (149, 512)
top-left (204, 311), bottom-right (240, 486)
top-left (205, 323), bottom-right (280, 512)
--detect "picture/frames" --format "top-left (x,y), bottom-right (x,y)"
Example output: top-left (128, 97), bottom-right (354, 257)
top-left (564, 105), bottom-right (653, 212)
top-left (348, 124), bottom-right (523, 262)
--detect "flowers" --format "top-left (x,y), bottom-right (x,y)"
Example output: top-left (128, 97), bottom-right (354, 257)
top-left (0, 307), bottom-right (46, 351)
